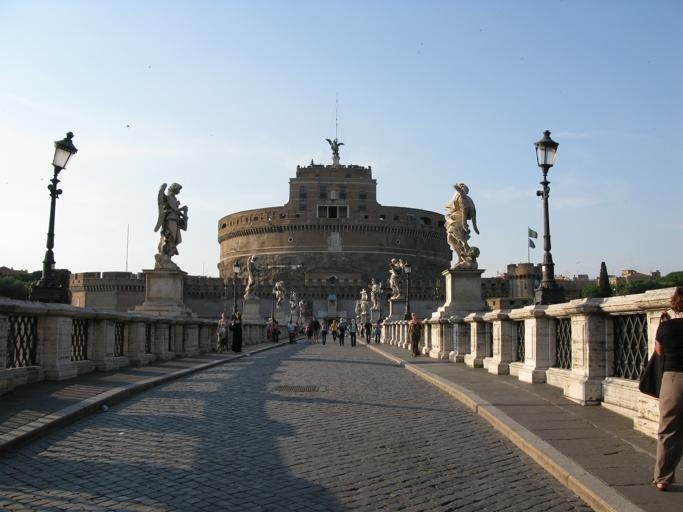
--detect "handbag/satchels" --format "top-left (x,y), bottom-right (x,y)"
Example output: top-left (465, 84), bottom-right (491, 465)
top-left (639, 352), bottom-right (664, 397)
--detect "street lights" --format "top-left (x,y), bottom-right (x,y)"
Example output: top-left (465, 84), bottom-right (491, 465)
top-left (400, 260), bottom-right (416, 323)
top-left (31, 128), bottom-right (77, 301)
top-left (532, 131), bottom-right (560, 306)
top-left (229, 258), bottom-right (247, 323)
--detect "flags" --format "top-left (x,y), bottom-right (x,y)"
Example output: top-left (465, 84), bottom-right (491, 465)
top-left (529, 238), bottom-right (535, 248)
top-left (527, 226), bottom-right (537, 239)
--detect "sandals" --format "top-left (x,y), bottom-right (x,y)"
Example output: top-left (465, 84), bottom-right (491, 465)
top-left (650, 478), bottom-right (666, 490)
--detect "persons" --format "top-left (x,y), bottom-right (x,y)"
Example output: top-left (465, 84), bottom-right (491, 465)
top-left (162, 182), bottom-right (188, 259)
top-left (653, 287), bottom-right (683, 490)
top-left (298, 298), bottom-right (304, 318)
top-left (231, 310), bottom-right (242, 354)
top-left (242, 254), bottom-right (264, 300)
top-left (264, 315), bottom-right (383, 348)
top-left (289, 292), bottom-right (298, 314)
top-left (273, 279), bottom-right (287, 310)
top-left (407, 312), bottom-right (423, 357)
top-left (368, 277), bottom-right (380, 310)
top-left (388, 257), bottom-right (404, 299)
top-left (354, 300), bottom-right (360, 317)
top-left (216, 311), bottom-right (229, 354)
top-left (443, 179), bottom-right (479, 270)
top-left (359, 288), bottom-right (368, 315)
